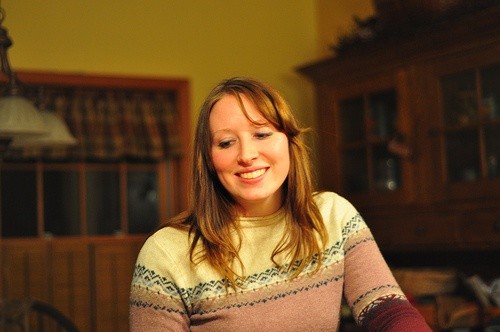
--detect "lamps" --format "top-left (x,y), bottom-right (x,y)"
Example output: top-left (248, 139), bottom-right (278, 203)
top-left (0, 7), bottom-right (79, 150)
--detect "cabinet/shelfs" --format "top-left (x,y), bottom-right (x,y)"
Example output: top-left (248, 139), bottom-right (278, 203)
top-left (296, 5), bottom-right (500, 254)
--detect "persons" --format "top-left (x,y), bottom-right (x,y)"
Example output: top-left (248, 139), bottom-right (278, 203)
top-left (126, 76), bottom-right (434, 332)
